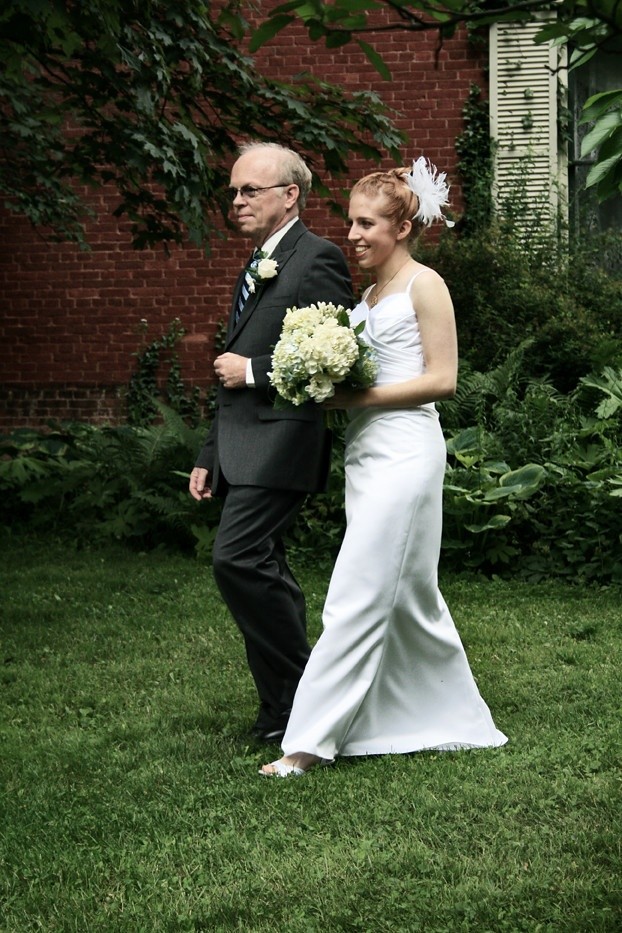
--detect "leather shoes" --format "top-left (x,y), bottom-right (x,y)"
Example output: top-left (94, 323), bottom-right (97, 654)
top-left (236, 709), bottom-right (290, 745)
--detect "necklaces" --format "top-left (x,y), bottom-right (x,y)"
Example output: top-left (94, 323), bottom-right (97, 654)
top-left (370, 256), bottom-right (412, 309)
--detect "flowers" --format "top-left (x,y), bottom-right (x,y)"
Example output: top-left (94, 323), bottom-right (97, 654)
top-left (266, 301), bottom-right (377, 411)
top-left (246, 246), bottom-right (279, 293)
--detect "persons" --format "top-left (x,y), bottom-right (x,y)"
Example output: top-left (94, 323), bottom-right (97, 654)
top-left (188, 145), bottom-right (360, 759)
top-left (258, 164), bottom-right (506, 778)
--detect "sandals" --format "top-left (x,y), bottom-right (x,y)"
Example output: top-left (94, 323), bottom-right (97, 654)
top-left (258, 753), bottom-right (336, 777)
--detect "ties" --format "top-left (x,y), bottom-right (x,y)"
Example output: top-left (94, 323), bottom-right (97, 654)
top-left (233, 249), bottom-right (263, 335)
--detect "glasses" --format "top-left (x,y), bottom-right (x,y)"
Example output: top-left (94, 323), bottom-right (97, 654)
top-left (224, 185), bottom-right (290, 201)
